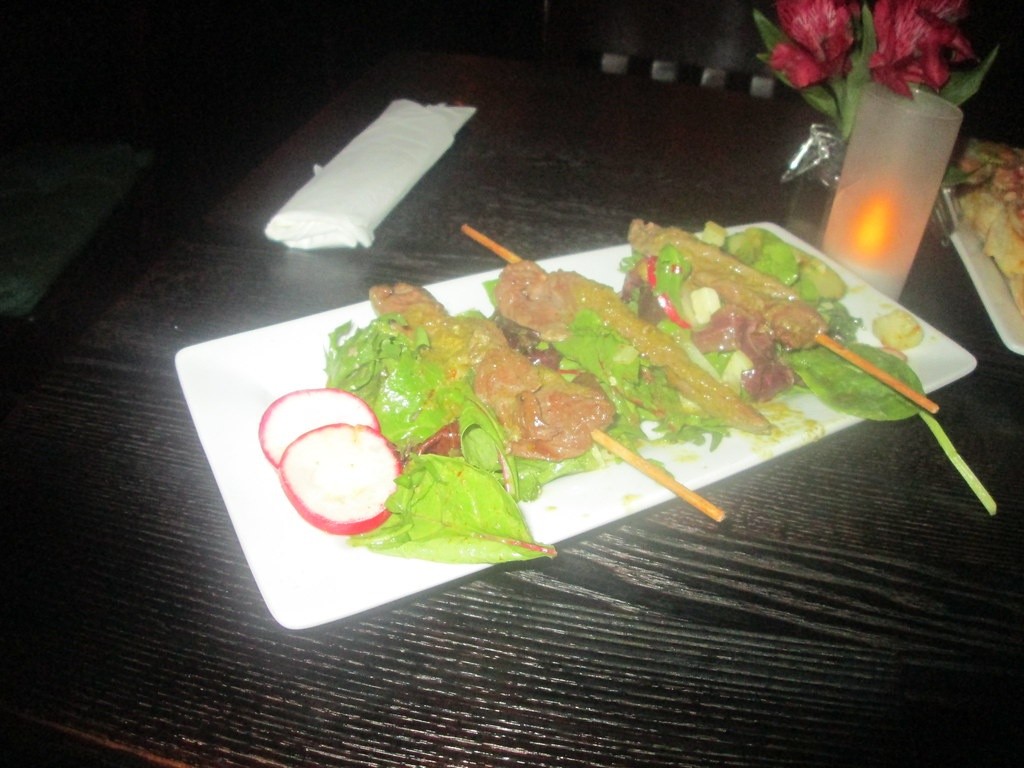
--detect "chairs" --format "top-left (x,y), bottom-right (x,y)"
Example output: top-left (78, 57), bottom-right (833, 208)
top-left (544, 0), bottom-right (782, 99)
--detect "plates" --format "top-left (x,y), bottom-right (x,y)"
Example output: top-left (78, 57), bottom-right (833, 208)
top-left (934, 187), bottom-right (1024, 356)
top-left (176, 221), bottom-right (977, 630)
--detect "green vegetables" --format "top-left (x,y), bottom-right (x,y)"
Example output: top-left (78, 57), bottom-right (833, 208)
top-left (321, 226), bottom-right (996, 571)
top-left (940, 136), bottom-right (1001, 185)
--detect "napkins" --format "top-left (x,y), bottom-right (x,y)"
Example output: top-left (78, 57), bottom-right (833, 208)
top-left (265, 98), bottom-right (476, 250)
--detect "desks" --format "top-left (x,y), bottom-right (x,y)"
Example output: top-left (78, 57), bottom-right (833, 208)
top-left (0, 76), bottom-right (1024, 768)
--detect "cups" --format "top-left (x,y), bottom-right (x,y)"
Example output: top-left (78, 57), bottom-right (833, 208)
top-left (820, 82), bottom-right (963, 302)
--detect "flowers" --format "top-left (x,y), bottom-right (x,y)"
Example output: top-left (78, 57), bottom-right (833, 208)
top-left (754, 0), bottom-right (1003, 188)
